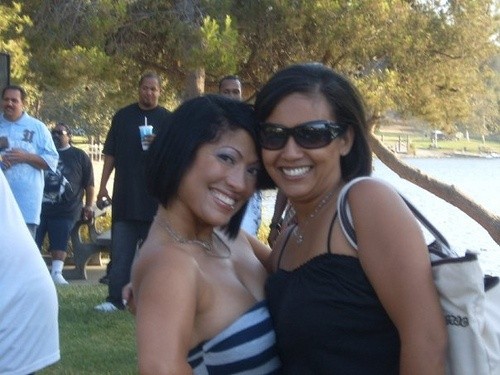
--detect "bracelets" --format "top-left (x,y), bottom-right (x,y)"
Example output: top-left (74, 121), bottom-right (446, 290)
top-left (269, 222), bottom-right (281, 229)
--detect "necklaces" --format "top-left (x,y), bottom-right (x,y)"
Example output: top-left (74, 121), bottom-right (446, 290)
top-left (293, 180), bottom-right (343, 245)
top-left (154, 215), bottom-right (232, 258)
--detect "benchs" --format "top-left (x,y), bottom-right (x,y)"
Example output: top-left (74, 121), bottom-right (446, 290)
top-left (71, 206), bottom-right (111, 284)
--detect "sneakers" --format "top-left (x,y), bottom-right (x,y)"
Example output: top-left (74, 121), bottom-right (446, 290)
top-left (93, 301), bottom-right (119, 312)
top-left (53, 273), bottom-right (69, 285)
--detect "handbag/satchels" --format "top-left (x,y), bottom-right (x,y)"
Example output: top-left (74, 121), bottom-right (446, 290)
top-left (337, 176), bottom-right (500, 375)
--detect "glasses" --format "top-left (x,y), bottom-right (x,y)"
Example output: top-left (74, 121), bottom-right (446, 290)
top-left (257, 119), bottom-right (347, 150)
top-left (52, 130), bottom-right (67, 136)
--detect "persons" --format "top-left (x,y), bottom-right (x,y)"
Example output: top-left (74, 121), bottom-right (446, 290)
top-left (130, 94), bottom-right (280, 375)
top-left (34, 123), bottom-right (95, 286)
top-left (268, 186), bottom-right (291, 250)
top-left (0, 85), bottom-right (60, 239)
top-left (0, 164), bottom-right (61, 375)
top-left (219, 75), bottom-right (263, 237)
top-left (122, 62), bottom-right (451, 375)
top-left (93, 72), bottom-right (172, 312)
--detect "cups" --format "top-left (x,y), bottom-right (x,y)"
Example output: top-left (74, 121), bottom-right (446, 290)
top-left (139, 125), bottom-right (153, 151)
